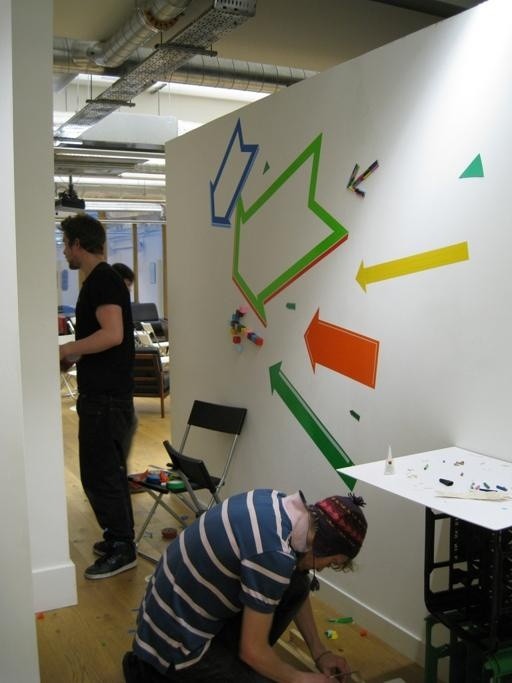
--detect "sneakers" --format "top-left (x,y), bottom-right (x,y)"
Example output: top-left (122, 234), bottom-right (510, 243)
top-left (85, 541), bottom-right (137, 579)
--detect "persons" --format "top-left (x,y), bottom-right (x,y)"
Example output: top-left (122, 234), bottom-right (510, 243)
top-left (59, 211), bottom-right (140, 582)
top-left (112, 262), bottom-right (134, 288)
top-left (121, 486), bottom-right (370, 682)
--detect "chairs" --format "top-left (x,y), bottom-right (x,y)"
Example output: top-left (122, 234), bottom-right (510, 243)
top-left (59, 300), bottom-right (171, 419)
top-left (126, 398), bottom-right (248, 569)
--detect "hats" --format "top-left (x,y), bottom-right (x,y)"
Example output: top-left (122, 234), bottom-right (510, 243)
top-left (307, 493), bottom-right (368, 591)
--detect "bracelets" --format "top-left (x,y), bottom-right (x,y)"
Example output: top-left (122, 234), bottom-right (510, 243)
top-left (315, 650), bottom-right (332, 663)
